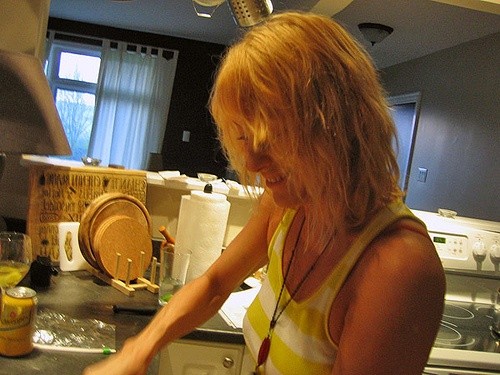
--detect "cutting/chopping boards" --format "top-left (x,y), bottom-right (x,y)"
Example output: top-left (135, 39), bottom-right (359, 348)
top-left (58, 222), bottom-right (88, 271)
top-left (78, 192), bottom-right (153, 282)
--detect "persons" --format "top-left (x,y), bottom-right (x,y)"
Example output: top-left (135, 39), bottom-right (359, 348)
top-left (81, 11), bottom-right (446, 375)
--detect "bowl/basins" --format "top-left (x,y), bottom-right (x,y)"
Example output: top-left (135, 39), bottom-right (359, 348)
top-left (198, 173), bottom-right (218, 183)
top-left (81, 157), bottom-right (101, 166)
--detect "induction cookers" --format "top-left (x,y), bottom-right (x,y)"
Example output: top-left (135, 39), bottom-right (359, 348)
top-left (415, 214), bottom-right (500, 372)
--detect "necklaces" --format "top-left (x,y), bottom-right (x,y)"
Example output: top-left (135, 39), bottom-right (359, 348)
top-left (250, 215), bottom-right (337, 375)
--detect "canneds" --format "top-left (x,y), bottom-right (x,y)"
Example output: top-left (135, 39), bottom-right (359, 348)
top-left (0, 286), bottom-right (38, 357)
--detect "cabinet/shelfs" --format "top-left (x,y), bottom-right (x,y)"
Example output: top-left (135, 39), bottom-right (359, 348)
top-left (158, 338), bottom-right (260, 375)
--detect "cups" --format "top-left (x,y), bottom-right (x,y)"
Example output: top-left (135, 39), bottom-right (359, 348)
top-left (158, 247), bottom-right (192, 307)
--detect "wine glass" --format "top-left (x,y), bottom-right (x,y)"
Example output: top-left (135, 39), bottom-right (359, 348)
top-left (0, 232), bottom-right (32, 296)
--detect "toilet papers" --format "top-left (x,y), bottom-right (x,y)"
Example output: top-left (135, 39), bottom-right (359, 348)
top-left (171, 190), bottom-right (232, 283)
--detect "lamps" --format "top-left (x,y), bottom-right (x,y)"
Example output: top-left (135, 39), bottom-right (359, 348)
top-left (358, 22), bottom-right (394, 46)
top-left (192, 0), bottom-right (273, 27)
